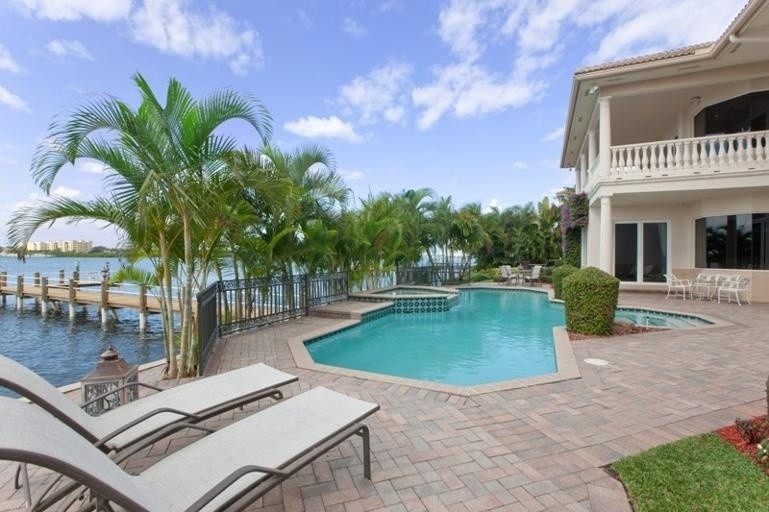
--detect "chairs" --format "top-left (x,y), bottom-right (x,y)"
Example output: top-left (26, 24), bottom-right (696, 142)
top-left (663, 271), bottom-right (751, 307)
top-left (498, 264), bottom-right (543, 288)
